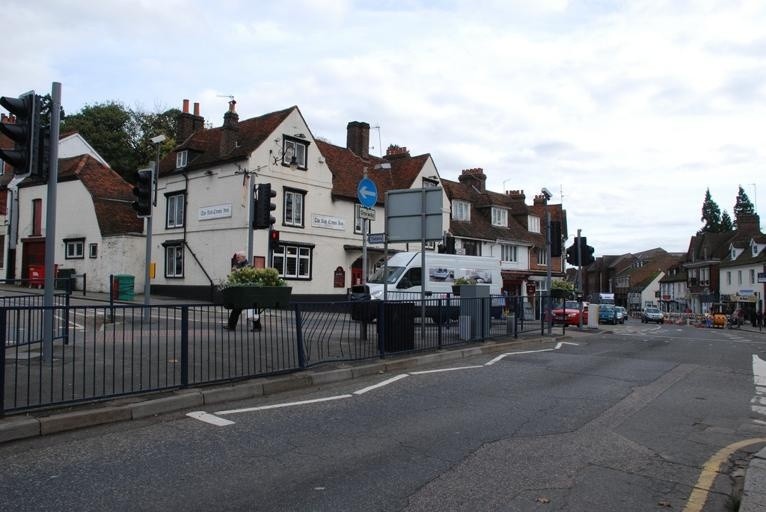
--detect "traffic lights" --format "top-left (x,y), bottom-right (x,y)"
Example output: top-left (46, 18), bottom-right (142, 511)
top-left (254, 183), bottom-right (276, 229)
top-left (0, 90), bottom-right (35, 179)
top-left (132, 168), bottom-right (153, 218)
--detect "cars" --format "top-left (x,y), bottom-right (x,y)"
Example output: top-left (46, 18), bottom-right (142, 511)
top-left (640, 307), bottom-right (664, 324)
top-left (542, 301), bottom-right (628, 327)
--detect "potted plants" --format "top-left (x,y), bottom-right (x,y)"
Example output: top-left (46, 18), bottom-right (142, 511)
top-left (215, 265), bottom-right (293, 309)
top-left (452, 277), bottom-right (478, 296)
top-left (550, 280), bottom-right (575, 298)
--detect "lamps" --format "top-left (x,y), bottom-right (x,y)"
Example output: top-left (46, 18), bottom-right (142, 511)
top-left (269, 145), bottom-right (299, 172)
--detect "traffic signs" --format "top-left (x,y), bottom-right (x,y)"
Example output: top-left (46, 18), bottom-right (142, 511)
top-left (359, 207), bottom-right (376, 220)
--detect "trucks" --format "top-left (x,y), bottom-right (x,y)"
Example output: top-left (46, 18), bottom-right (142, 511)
top-left (591, 293), bottom-right (616, 307)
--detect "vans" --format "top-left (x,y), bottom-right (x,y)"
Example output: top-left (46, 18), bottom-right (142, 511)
top-left (347, 250), bottom-right (504, 326)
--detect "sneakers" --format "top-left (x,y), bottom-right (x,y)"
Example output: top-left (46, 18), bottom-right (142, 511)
top-left (252, 328), bottom-right (261, 332)
top-left (223, 325), bottom-right (236, 331)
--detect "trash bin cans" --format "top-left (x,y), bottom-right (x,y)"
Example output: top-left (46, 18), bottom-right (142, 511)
top-left (351, 285), bottom-right (371, 320)
top-left (113, 275), bottom-right (135, 301)
top-left (378, 301), bottom-right (415, 351)
top-left (57, 269), bottom-right (76, 291)
top-left (28, 265), bottom-right (45, 289)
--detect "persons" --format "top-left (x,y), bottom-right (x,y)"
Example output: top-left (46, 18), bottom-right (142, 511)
top-left (749, 307), bottom-right (766, 331)
top-left (218, 250), bottom-right (263, 333)
top-left (512, 288), bottom-right (521, 317)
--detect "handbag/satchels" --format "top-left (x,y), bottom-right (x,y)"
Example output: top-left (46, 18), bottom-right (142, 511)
top-left (248, 308), bottom-right (259, 321)
